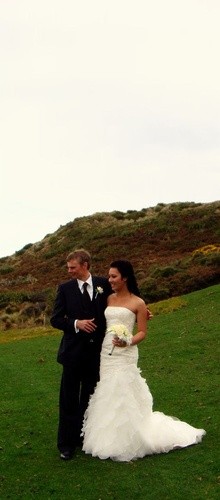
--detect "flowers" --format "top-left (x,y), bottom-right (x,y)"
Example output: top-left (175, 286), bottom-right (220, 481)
top-left (94, 287), bottom-right (103, 298)
top-left (105, 324), bottom-right (133, 356)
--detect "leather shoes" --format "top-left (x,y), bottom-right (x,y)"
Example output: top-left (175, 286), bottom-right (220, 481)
top-left (60, 447), bottom-right (71, 460)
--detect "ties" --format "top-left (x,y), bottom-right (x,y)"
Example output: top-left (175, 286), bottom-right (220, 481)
top-left (82, 283), bottom-right (91, 302)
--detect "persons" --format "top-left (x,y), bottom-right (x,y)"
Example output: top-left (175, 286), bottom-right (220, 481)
top-left (51, 249), bottom-right (153, 460)
top-left (80, 260), bottom-right (206, 463)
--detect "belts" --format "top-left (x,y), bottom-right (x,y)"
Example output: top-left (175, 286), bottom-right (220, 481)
top-left (87, 338), bottom-right (97, 344)
